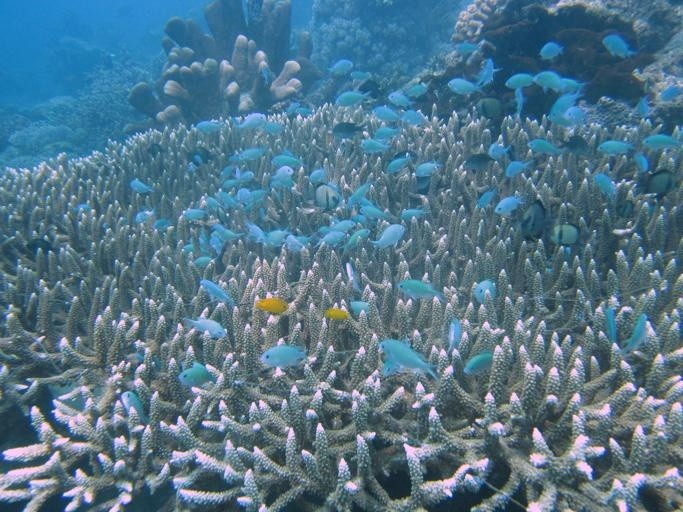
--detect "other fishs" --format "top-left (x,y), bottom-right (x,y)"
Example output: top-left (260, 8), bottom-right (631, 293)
top-left (72, 33), bottom-right (683, 425)
